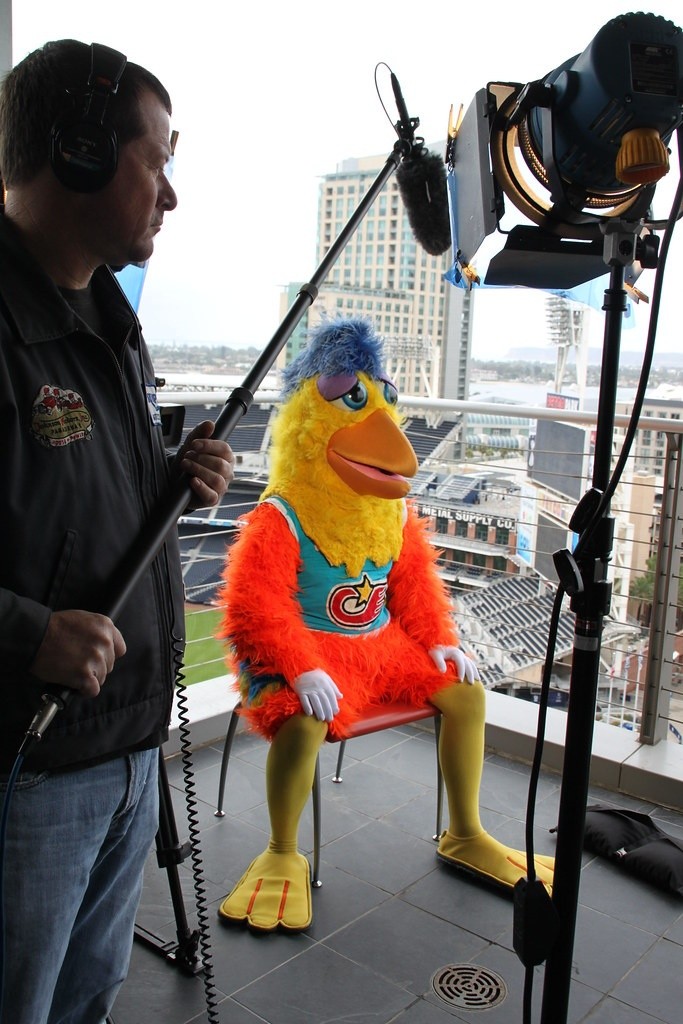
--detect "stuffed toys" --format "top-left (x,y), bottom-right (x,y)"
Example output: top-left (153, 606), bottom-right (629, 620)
top-left (213, 320), bottom-right (556, 938)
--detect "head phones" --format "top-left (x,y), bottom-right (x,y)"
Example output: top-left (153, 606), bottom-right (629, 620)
top-left (52, 43), bottom-right (126, 194)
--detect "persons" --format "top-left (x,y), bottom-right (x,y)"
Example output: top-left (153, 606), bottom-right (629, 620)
top-left (0, 39), bottom-right (237, 1024)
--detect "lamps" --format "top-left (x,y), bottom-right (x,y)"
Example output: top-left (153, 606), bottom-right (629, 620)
top-left (491, 5), bottom-right (683, 1024)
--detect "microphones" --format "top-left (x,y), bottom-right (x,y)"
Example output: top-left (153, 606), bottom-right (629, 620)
top-left (391, 73), bottom-right (451, 257)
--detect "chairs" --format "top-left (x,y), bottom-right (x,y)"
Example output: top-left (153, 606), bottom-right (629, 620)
top-left (213, 509), bottom-right (446, 890)
